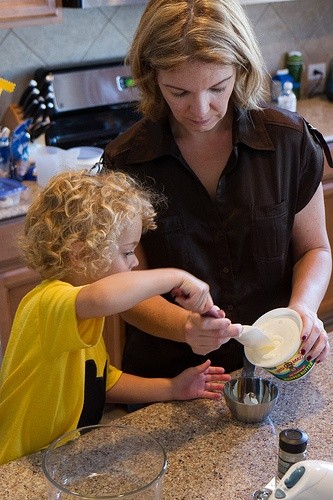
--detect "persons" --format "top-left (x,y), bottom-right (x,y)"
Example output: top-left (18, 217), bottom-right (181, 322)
top-left (0, 169), bottom-right (231, 465)
top-left (96, 0), bottom-right (333, 414)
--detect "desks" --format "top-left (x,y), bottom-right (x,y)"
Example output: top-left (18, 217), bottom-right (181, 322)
top-left (0, 328), bottom-right (333, 500)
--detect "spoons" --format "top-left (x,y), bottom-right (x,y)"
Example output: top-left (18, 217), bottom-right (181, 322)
top-left (169, 287), bottom-right (275, 355)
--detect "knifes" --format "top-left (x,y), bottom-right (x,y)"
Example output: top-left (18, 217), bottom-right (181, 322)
top-left (17, 74), bottom-right (56, 121)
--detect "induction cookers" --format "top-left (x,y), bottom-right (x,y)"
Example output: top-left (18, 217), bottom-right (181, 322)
top-left (37, 57), bottom-right (143, 150)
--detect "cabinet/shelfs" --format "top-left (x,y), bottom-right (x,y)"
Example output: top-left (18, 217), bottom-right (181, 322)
top-left (61, 0), bottom-right (146, 9)
top-left (317, 141), bottom-right (333, 325)
top-left (0, 215), bottom-right (125, 371)
top-left (0, 0), bottom-right (64, 30)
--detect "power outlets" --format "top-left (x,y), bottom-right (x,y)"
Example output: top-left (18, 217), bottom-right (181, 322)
top-left (306, 63), bottom-right (327, 80)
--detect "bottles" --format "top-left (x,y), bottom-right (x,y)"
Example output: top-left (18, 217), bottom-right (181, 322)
top-left (279, 81), bottom-right (297, 113)
top-left (286, 49), bottom-right (303, 99)
top-left (277, 429), bottom-right (309, 480)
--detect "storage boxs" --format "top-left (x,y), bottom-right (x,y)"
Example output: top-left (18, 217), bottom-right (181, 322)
top-left (0, 177), bottom-right (29, 209)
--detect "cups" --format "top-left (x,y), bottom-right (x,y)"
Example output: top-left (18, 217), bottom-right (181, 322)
top-left (42, 424), bottom-right (169, 500)
top-left (33, 142), bottom-right (103, 188)
top-left (244, 306), bottom-right (318, 383)
top-left (222, 376), bottom-right (279, 426)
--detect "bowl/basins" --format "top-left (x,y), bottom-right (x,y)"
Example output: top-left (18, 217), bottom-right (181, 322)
top-left (0, 177), bottom-right (24, 208)
